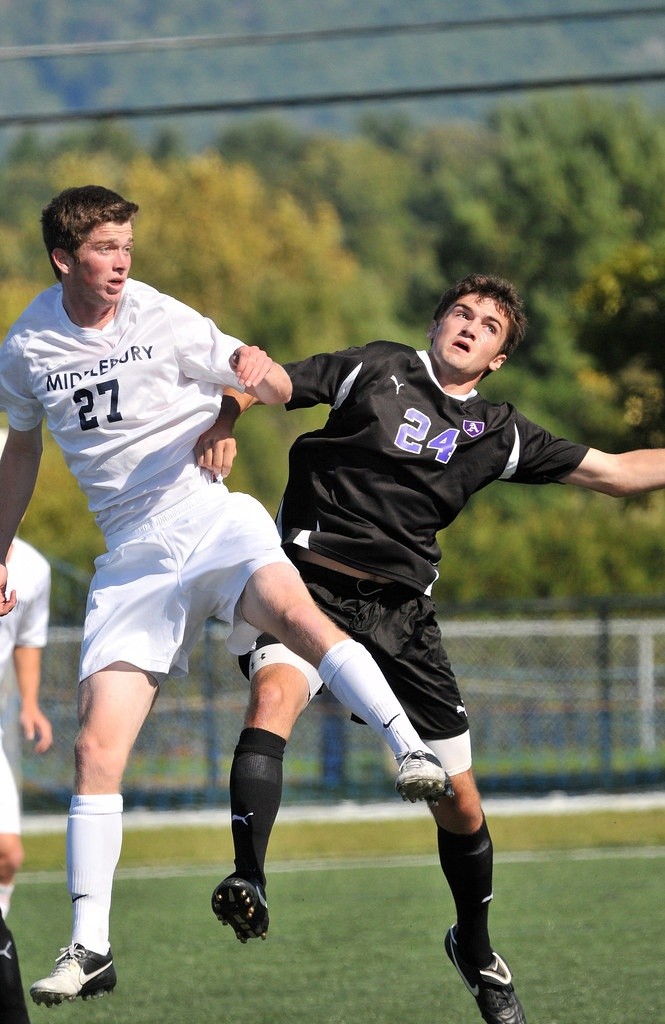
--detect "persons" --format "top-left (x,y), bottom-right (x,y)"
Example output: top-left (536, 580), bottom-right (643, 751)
top-left (189, 276), bottom-right (663, 1024)
top-left (3, 184), bottom-right (461, 1009)
top-left (1, 535), bottom-right (56, 937)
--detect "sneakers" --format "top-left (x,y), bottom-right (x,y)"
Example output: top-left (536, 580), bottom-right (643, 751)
top-left (30, 939), bottom-right (117, 1009)
top-left (440, 921), bottom-right (528, 1024)
top-left (209, 873), bottom-right (271, 944)
top-left (394, 750), bottom-right (454, 809)
